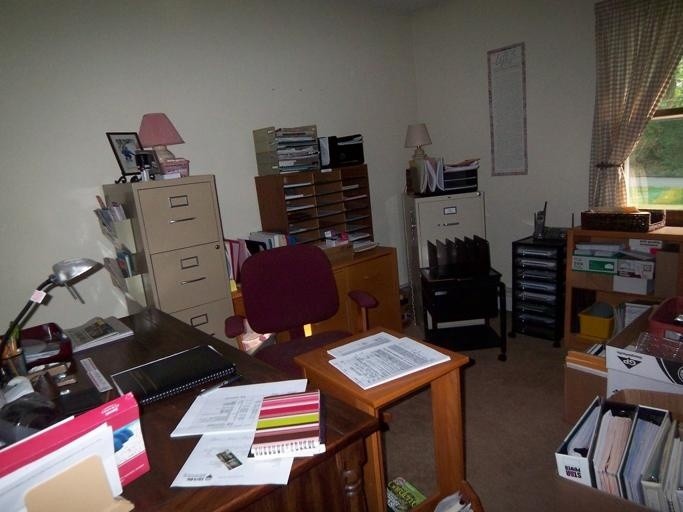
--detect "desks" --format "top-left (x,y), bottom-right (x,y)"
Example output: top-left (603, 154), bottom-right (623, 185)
top-left (22, 304), bottom-right (385, 512)
top-left (231, 244), bottom-right (403, 347)
top-left (292, 323), bottom-right (485, 512)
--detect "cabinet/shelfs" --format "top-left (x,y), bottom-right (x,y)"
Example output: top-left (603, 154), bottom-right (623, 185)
top-left (508, 236), bottom-right (566, 348)
top-left (563, 225), bottom-right (683, 350)
top-left (402, 192), bottom-right (487, 326)
top-left (254, 163), bottom-right (374, 246)
top-left (101, 174), bottom-right (239, 351)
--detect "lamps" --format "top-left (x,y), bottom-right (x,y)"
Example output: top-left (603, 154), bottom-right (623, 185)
top-left (134, 111), bottom-right (187, 165)
top-left (0, 256), bottom-right (99, 381)
top-left (403, 122), bottom-right (432, 173)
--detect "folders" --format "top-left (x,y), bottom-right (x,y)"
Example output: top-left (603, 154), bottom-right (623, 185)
top-left (640, 416), bottom-right (678, 512)
top-left (661, 422), bottom-right (683, 511)
top-left (593, 399), bottom-right (638, 500)
top-left (554, 395), bottom-right (601, 488)
top-left (620, 403), bottom-right (670, 504)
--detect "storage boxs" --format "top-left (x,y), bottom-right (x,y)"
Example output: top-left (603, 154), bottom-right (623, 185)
top-left (606, 304), bottom-right (683, 400)
top-left (577, 305), bottom-right (614, 339)
top-left (553, 388), bottom-right (683, 512)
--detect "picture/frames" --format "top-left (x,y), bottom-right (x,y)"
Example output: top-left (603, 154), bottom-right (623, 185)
top-left (105, 132), bottom-right (156, 185)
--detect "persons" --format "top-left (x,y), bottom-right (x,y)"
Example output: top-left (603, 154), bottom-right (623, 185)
top-left (139, 156), bottom-right (145, 172)
top-left (122, 147), bottom-right (135, 162)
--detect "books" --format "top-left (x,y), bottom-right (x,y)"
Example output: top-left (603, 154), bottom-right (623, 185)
top-left (65, 316), bottom-right (134, 353)
top-left (586, 343), bottom-right (606, 358)
top-left (248, 388), bottom-right (326, 459)
top-left (248, 230), bottom-right (287, 250)
top-left (446, 159), bottom-right (479, 171)
top-left (409, 157), bottom-right (445, 193)
top-left (388, 476), bottom-right (426, 512)
top-left (109, 346), bottom-right (236, 408)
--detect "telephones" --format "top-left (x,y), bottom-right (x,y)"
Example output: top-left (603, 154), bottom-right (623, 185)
top-left (533, 200), bottom-right (548, 240)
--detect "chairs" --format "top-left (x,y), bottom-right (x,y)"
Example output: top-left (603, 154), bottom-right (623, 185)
top-left (223, 242), bottom-right (379, 382)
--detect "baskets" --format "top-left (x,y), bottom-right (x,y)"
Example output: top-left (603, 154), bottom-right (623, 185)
top-left (581, 208), bottom-right (666, 232)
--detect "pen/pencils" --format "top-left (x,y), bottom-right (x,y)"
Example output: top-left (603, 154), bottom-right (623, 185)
top-left (199, 374), bottom-right (244, 397)
top-left (0, 320), bottom-right (28, 377)
